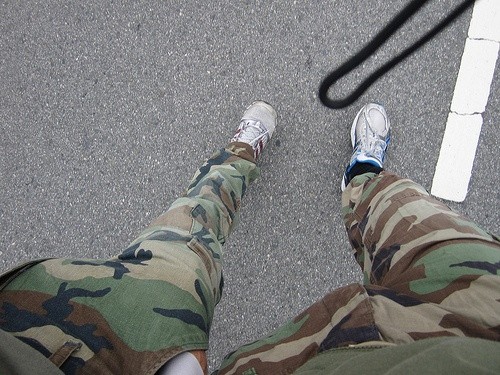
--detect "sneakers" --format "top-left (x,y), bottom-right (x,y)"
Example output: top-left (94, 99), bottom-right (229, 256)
top-left (338, 104), bottom-right (389, 192)
top-left (232, 102), bottom-right (278, 159)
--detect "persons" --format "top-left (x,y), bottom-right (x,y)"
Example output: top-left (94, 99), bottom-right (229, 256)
top-left (0, 99), bottom-right (500, 374)
